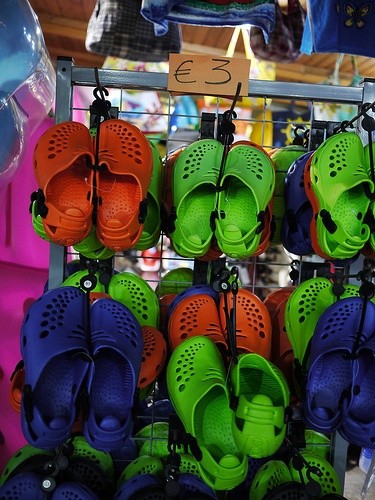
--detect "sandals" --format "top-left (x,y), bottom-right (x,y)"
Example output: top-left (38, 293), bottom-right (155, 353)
top-left (30, 118), bottom-right (162, 263)
top-left (263, 277), bottom-right (375, 447)
top-left (0, 399), bottom-right (351, 500)
top-left (160, 140), bottom-right (276, 260)
top-left (155, 268), bottom-right (291, 490)
top-left (9, 265), bottom-right (166, 450)
top-left (270, 132), bottom-right (375, 261)
